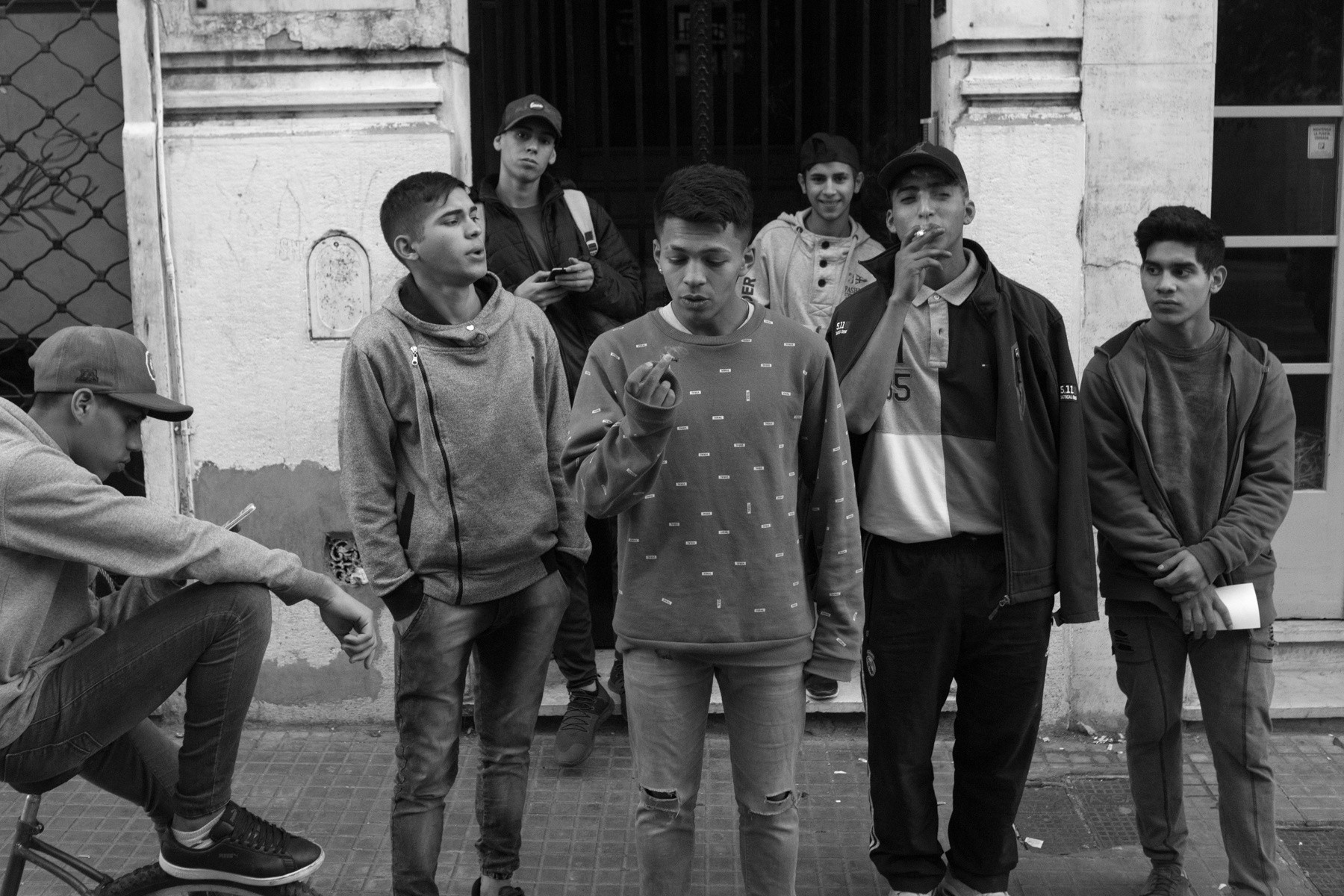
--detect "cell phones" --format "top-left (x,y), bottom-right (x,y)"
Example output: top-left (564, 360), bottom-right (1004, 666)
top-left (546, 265), bottom-right (575, 282)
top-left (221, 502), bottom-right (256, 529)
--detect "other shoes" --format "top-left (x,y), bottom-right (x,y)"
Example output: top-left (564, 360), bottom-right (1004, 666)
top-left (472, 878), bottom-right (524, 896)
top-left (889, 886), bottom-right (936, 896)
top-left (942, 877), bottom-right (1010, 896)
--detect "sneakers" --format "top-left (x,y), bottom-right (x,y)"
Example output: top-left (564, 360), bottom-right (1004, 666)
top-left (554, 681), bottom-right (613, 767)
top-left (803, 671), bottom-right (837, 699)
top-left (1137, 865), bottom-right (1189, 896)
top-left (158, 800), bottom-right (325, 887)
top-left (608, 660), bottom-right (628, 724)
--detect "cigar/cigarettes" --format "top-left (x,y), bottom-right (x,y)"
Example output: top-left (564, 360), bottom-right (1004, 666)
top-left (661, 354), bottom-right (678, 362)
top-left (914, 230), bottom-right (925, 237)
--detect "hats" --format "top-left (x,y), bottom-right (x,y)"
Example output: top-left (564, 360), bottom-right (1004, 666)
top-left (27, 325), bottom-right (194, 421)
top-left (877, 143), bottom-right (969, 198)
top-left (801, 133), bottom-right (860, 173)
top-left (499, 94), bottom-right (564, 140)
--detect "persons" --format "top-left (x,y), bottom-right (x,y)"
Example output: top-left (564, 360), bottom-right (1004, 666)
top-left (0, 325), bottom-right (376, 896)
top-left (560, 162), bottom-right (868, 896)
top-left (339, 172), bottom-right (591, 896)
top-left (1078, 205), bottom-right (1296, 896)
top-left (825, 142), bottom-right (1099, 896)
top-left (734, 133), bottom-right (887, 700)
top-left (469, 92), bottom-right (647, 754)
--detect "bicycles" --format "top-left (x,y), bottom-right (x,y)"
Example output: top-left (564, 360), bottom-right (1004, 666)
top-left (0, 766), bottom-right (318, 896)
